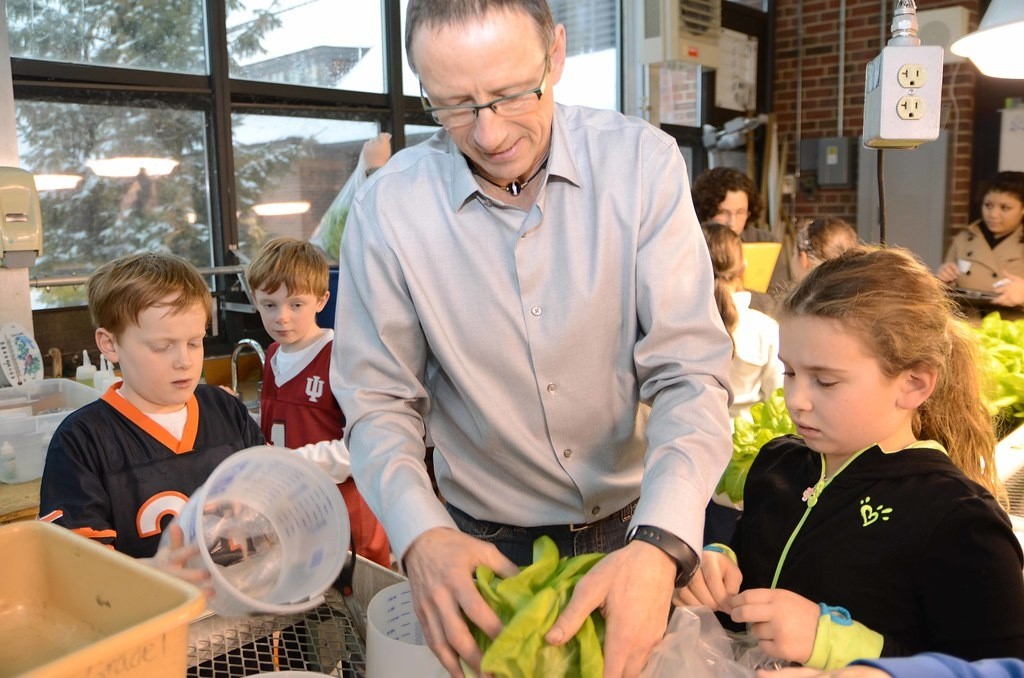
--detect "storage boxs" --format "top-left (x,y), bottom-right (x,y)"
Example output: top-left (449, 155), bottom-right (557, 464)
top-left (1, 519), bottom-right (206, 678)
top-left (0, 378), bottom-right (104, 484)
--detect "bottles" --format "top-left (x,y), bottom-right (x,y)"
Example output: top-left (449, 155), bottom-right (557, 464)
top-left (76, 348), bottom-right (122, 393)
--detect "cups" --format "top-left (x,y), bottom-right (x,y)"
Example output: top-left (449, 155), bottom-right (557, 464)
top-left (366, 580), bottom-right (450, 678)
top-left (157, 445), bottom-right (350, 618)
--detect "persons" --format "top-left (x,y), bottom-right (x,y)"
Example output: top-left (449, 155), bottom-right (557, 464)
top-left (217, 238), bottom-right (398, 678)
top-left (37, 250), bottom-right (276, 678)
top-left (329, 0), bottom-right (1024, 678)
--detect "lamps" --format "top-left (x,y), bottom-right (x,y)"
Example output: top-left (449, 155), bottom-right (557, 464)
top-left (949, 0), bottom-right (1024, 82)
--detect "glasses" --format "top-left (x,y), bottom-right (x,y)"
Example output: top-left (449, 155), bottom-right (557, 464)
top-left (419, 54), bottom-right (550, 128)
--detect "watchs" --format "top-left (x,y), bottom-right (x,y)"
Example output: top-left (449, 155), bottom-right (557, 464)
top-left (625, 525), bottom-right (700, 588)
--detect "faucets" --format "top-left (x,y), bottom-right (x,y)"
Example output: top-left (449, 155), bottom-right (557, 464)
top-left (230, 339), bottom-right (266, 403)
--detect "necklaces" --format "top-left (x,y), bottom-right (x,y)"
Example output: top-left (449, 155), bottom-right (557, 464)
top-left (469, 156), bottom-right (549, 196)
top-left (801, 476), bottom-right (829, 501)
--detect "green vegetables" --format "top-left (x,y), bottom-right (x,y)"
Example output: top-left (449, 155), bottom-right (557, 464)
top-left (715, 385), bottom-right (806, 504)
top-left (950, 310), bottom-right (1024, 422)
top-left (466, 534), bottom-right (617, 678)
